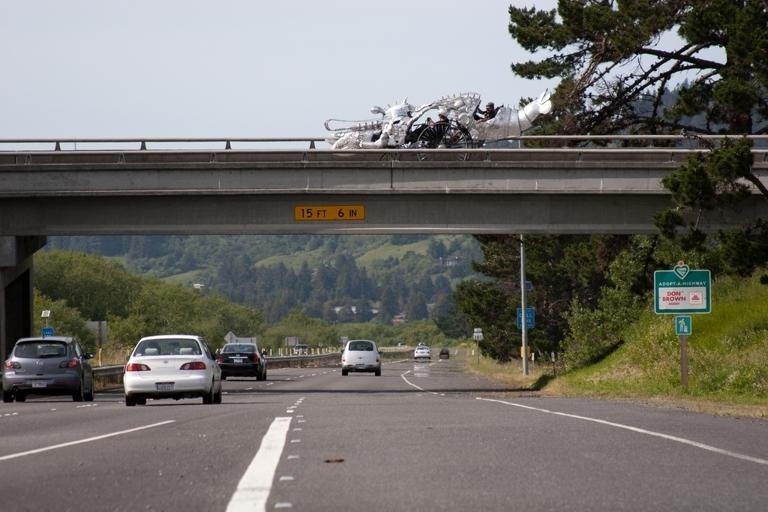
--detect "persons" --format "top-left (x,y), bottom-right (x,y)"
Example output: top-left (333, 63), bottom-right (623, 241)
top-left (474, 101), bottom-right (504, 122)
top-left (405, 109), bottom-right (449, 142)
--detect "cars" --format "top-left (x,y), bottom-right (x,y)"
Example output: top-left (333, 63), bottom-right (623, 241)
top-left (414, 346), bottom-right (431, 359)
top-left (341, 340), bottom-right (381, 376)
top-left (290, 344), bottom-right (312, 355)
top-left (439, 348), bottom-right (449, 359)
top-left (123, 332), bottom-right (267, 406)
top-left (2, 336), bottom-right (94, 403)
